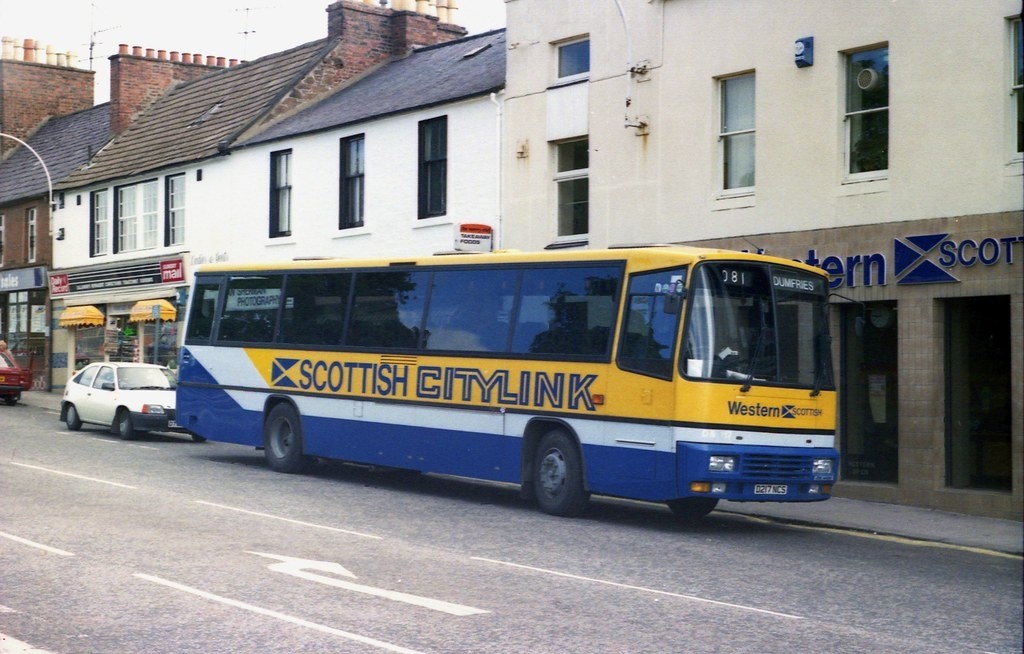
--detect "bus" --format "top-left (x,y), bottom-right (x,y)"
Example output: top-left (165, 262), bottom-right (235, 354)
top-left (176, 244), bottom-right (866, 521)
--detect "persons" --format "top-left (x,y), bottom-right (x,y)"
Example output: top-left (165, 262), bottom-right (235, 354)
top-left (717, 337), bottom-right (741, 379)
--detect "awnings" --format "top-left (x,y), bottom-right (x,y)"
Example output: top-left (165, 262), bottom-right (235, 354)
top-left (59, 305), bottom-right (105, 326)
top-left (129, 300), bottom-right (178, 321)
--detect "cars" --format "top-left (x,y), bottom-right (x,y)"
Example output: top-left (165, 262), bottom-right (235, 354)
top-left (60, 361), bottom-right (206, 443)
top-left (0, 340), bottom-right (32, 406)
